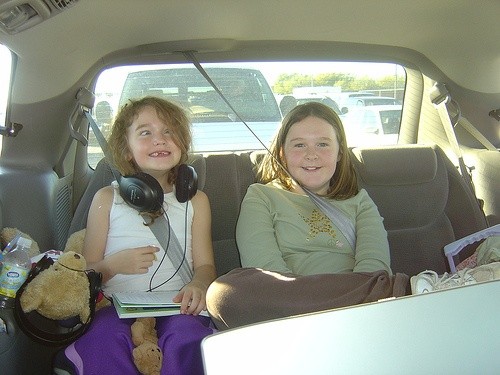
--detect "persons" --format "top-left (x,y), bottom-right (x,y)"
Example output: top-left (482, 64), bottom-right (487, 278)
top-left (206, 102), bottom-right (500, 332)
top-left (65, 98), bottom-right (216, 375)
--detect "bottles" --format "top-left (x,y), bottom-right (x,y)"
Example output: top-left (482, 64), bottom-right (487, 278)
top-left (0, 237), bottom-right (32, 310)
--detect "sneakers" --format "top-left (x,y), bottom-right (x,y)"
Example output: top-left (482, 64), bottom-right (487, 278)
top-left (410, 267), bottom-right (494, 298)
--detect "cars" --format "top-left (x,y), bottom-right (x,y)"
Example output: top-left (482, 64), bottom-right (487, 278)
top-left (95, 66), bottom-right (403, 159)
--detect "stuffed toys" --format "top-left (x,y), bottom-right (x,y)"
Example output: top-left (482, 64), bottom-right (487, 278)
top-left (131, 318), bottom-right (163, 375)
top-left (2, 227), bottom-right (91, 325)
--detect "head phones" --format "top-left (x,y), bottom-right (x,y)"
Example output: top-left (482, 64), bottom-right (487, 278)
top-left (14, 255), bottom-right (104, 344)
top-left (113, 164), bottom-right (198, 212)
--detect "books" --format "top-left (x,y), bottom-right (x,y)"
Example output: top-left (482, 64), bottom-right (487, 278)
top-left (111, 292), bottom-right (210, 319)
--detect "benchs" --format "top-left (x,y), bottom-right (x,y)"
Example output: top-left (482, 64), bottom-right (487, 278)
top-left (68, 144), bottom-right (487, 279)
